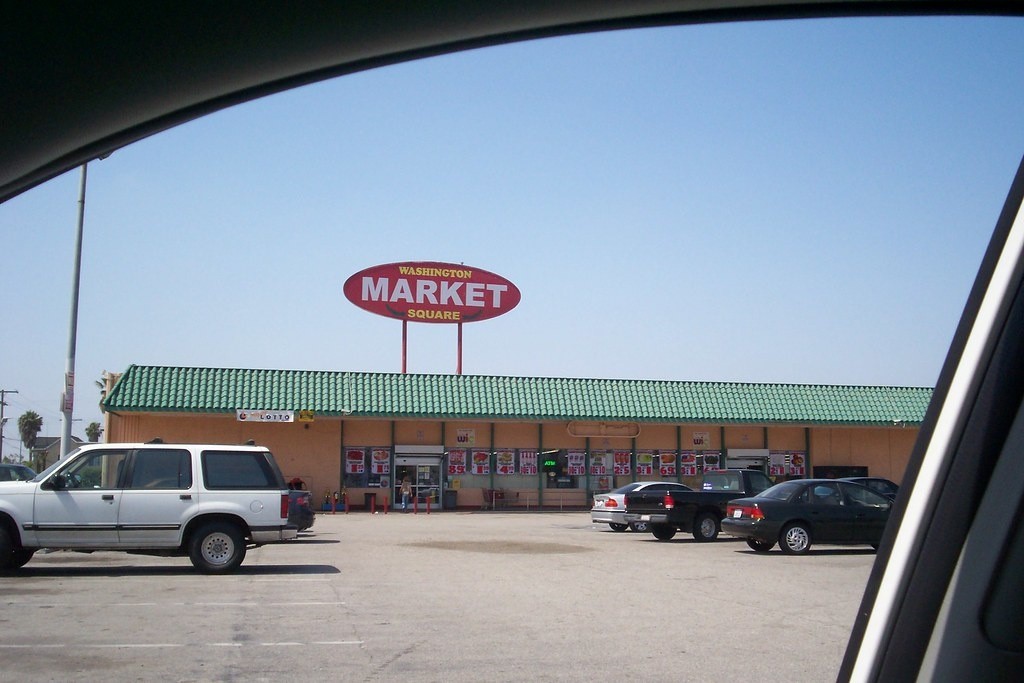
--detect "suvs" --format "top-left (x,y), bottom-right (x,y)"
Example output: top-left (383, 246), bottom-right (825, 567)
top-left (0, 438), bottom-right (317, 576)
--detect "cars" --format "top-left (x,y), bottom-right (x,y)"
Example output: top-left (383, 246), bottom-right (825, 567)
top-left (0, 463), bottom-right (37, 480)
top-left (721, 479), bottom-right (895, 555)
top-left (802, 476), bottom-right (899, 503)
top-left (590, 482), bottom-right (694, 533)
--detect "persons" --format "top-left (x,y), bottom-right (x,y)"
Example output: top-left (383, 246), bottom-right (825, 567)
top-left (399, 477), bottom-right (413, 513)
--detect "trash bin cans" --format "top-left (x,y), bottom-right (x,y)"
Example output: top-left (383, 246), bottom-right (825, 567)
top-left (364, 492), bottom-right (376, 512)
top-left (445, 490), bottom-right (458, 509)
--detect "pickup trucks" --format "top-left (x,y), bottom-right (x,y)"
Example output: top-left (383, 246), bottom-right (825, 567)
top-left (624, 469), bottom-right (779, 543)
